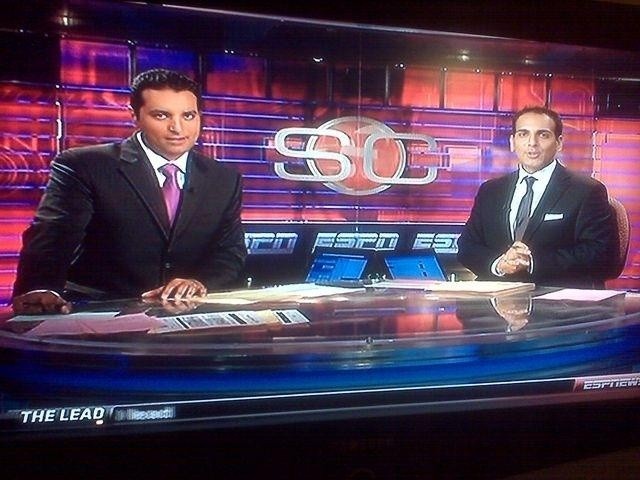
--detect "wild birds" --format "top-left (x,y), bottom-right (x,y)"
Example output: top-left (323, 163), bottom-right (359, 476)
top-left (376, 248), bottom-right (449, 281)
top-left (301, 246), bottom-right (375, 282)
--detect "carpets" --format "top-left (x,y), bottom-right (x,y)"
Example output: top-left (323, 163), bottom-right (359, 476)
top-left (160, 164), bottom-right (182, 227)
top-left (514, 177), bottom-right (536, 237)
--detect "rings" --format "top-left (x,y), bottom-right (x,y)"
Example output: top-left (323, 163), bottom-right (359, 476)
top-left (68, 300), bottom-right (89, 306)
top-left (508, 244), bottom-right (521, 250)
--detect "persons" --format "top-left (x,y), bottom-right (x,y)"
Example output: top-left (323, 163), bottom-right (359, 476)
top-left (452, 104), bottom-right (621, 285)
top-left (12, 66), bottom-right (248, 315)
top-left (454, 292), bottom-right (588, 337)
